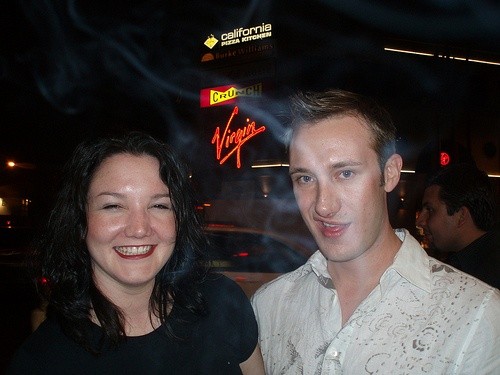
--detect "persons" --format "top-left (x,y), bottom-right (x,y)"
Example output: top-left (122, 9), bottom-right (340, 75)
top-left (0, 128), bottom-right (267, 375)
top-left (249, 87), bottom-right (500, 375)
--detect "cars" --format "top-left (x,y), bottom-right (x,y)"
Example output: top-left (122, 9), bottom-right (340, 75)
top-left (198, 223), bottom-right (313, 300)
